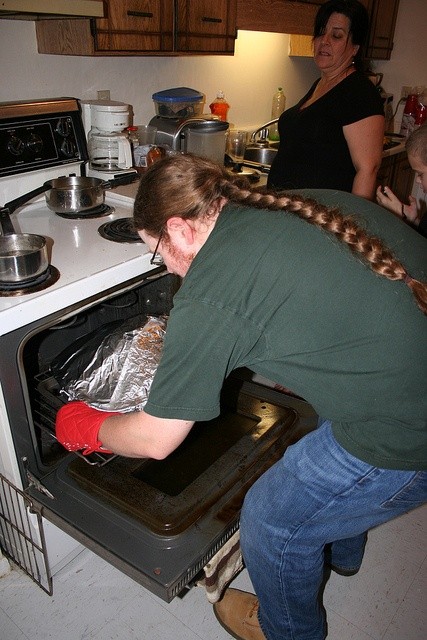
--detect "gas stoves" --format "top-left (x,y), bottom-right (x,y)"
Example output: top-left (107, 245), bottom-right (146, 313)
top-left (0, 194), bottom-right (158, 334)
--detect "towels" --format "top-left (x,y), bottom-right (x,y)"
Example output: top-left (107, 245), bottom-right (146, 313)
top-left (193, 529), bottom-right (245, 606)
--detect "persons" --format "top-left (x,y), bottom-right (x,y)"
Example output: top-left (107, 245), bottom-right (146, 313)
top-left (376, 128), bottom-right (427, 240)
top-left (55, 155), bottom-right (427, 639)
top-left (266, 1), bottom-right (387, 207)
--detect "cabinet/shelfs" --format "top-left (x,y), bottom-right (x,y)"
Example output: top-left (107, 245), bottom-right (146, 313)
top-left (33, 1), bottom-right (239, 58)
top-left (236, 0), bottom-right (323, 37)
top-left (288, 1), bottom-right (401, 61)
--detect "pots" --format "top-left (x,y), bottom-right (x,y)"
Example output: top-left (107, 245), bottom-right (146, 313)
top-left (40, 172), bottom-right (140, 215)
top-left (0, 206), bottom-right (55, 291)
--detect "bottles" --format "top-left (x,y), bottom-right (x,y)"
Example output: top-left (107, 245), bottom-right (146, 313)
top-left (268, 87), bottom-right (287, 141)
top-left (207, 90), bottom-right (230, 153)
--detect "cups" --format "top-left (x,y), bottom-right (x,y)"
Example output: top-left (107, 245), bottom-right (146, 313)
top-left (227, 129), bottom-right (247, 164)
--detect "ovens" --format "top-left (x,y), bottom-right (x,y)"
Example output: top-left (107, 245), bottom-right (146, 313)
top-left (0, 256), bottom-right (310, 606)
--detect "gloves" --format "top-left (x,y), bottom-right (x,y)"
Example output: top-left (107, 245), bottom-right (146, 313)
top-left (56, 401), bottom-right (121, 456)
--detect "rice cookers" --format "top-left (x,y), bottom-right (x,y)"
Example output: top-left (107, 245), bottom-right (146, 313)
top-left (79, 99), bottom-right (131, 138)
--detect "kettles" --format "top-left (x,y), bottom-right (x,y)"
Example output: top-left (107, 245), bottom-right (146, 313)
top-left (87, 126), bottom-right (133, 172)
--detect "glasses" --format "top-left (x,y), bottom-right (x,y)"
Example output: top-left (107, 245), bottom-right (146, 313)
top-left (150, 231), bottom-right (168, 266)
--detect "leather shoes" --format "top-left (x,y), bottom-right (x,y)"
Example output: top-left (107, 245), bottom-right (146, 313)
top-left (213, 587), bottom-right (265, 640)
